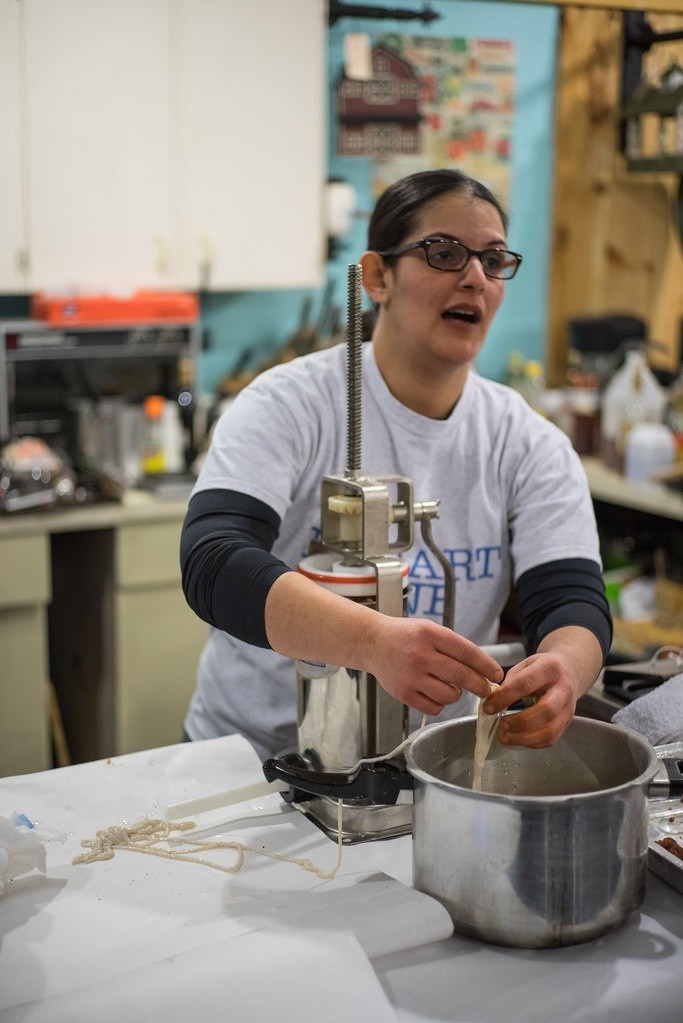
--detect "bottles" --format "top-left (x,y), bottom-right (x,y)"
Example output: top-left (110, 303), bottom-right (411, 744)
top-left (506, 348), bottom-right (669, 469)
top-left (133, 387), bottom-right (169, 491)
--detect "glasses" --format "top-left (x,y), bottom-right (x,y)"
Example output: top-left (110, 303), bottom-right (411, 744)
top-left (380, 239), bottom-right (525, 280)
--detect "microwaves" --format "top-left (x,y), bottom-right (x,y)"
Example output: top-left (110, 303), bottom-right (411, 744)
top-left (1, 318), bottom-right (201, 506)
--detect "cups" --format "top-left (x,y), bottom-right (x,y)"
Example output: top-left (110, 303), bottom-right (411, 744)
top-left (620, 421), bottom-right (678, 482)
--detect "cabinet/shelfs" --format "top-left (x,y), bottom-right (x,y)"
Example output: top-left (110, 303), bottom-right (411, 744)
top-left (109, 526), bottom-right (216, 760)
top-left (0, 533), bottom-right (51, 780)
top-left (0, 1), bottom-right (328, 296)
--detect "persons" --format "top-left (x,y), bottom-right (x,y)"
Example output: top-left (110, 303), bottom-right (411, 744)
top-left (181, 169), bottom-right (614, 776)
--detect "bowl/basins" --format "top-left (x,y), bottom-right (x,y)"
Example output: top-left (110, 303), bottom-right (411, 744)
top-left (402, 714), bottom-right (665, 949)
